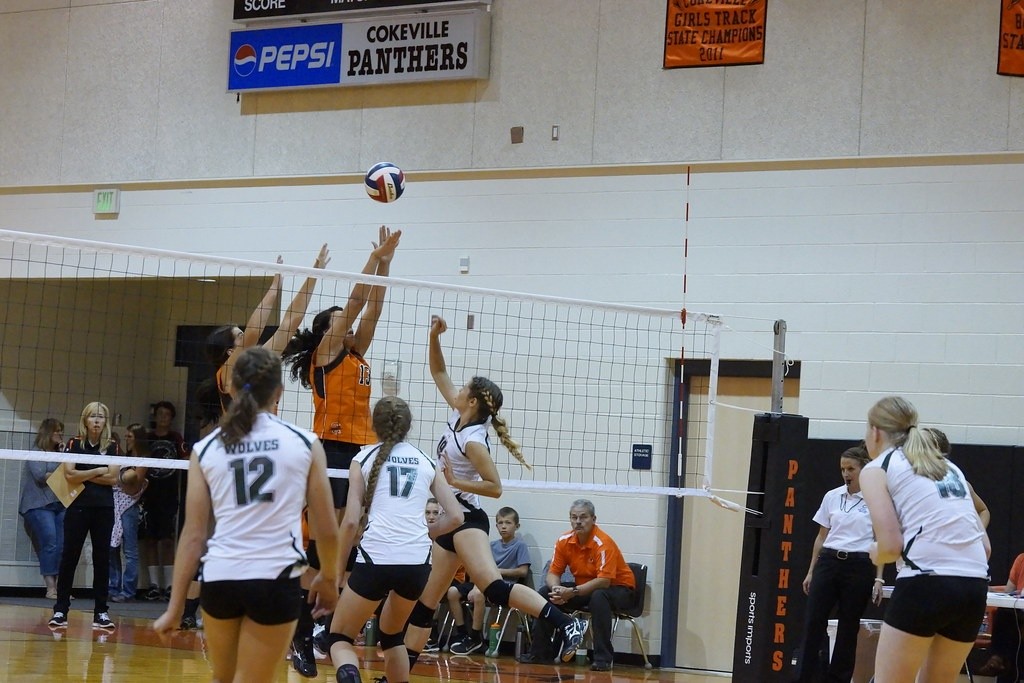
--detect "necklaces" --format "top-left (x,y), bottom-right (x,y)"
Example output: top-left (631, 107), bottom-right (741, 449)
top-left (840, 480), bottom-right (863, 513)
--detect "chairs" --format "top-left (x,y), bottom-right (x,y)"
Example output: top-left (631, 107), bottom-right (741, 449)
top-left (437, 560), bottom-right (652, 670)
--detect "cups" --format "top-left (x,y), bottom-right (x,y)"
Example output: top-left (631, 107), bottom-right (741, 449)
top-left (575, 647), bottom-right (587, 666)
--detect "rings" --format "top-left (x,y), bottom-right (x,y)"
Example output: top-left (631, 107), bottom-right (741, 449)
top-left (875, 593), bottom-right (878, 594)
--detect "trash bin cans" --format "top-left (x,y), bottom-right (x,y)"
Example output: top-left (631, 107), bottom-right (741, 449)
top-left (828, 619), bottom-right (881, 683)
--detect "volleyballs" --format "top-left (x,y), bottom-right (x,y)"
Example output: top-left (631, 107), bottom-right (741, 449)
top-left (365, 162), bottom-right (405, 203)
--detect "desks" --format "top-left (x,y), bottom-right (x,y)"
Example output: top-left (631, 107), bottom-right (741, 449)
top-left (869, 586), bottom-right (1024, 683)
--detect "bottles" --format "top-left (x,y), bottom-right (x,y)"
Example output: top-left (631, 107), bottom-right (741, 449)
top-left (490, 623), bottom-right (500, 658)
top-left (112, 413), bottom-right (122, 426)
top-left (515, 624), bottom-right (527, 660)
top-left (366, 614), bottom-right (376, 646)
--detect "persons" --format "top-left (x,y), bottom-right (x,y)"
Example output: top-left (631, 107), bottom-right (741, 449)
top-left (800, 447), bottom-right (885, 683)
top-left (328, 396), bottom-right (464, 683)
top-left (154, 347), bottom-right (339, 683)
top-left (374, 314), bottom-right (589, 683)
top-left (108, 423), bottom-right (150, 603)
top-left (139, 401), bottom-right (189, 602)
top-left (49, 402), bottom-right (120, 627)
top-left (516, 499), bottom-right (636, 671)
top-left (18, 418), bottom-right (75, 600)
top-left (282, 226), bottom-right (401, 678)
top-left (200, 244), bottom-right (331, 430)
top-left (924, 428), bottom-right (990, 529)
top-left (858, 396), bottom-right (990, 683)
top-left (423, 498), bottom-right (531, 655)
top-left (996, 551), bottom-right (1024, 683)
top-left (176, 562), bottom-right (204, 630)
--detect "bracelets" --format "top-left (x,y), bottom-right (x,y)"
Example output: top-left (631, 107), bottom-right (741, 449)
top-left (573, 586), bottom-right (579, 593)
top-left (875, 578), bottom-right (885, 584)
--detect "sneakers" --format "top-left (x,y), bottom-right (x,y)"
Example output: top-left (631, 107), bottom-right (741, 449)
top-left (450, 633), bottom-right (467, 649)
top-left (290, 634), bottom-right (317, 678)
top-left (287, 640), bottom-right (327, 660)
top-left (450, 633), bottom-right (483, 655)
top-left (422, 640), bottom-right (439, 652)
top-left (92, 627), bottom-right (116, 647)
top-left (92, 610), bottom-right (115, 628)
top-left (561, 617), bottom-right (589, 662)
top-left (49, 626), bottom-right (67, 642)
top-left (520, 652), bottom-right (548, 664)
top-left (49, 611), bottom-right (68, 626)
top-left (591, 648), bottom-right (615, 671)
top-left (179, 615), bottom-right (199, 630)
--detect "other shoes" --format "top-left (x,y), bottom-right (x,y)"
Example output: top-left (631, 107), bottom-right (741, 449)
top-left (372, 675), bottom-right (409, 683)
top-left (352, 633), bottom-right (365, 646)
top-left (988, 655), bottom-right (1004, 676)
top-left (165, 586), bottom-right (173, 600)
top-left (145, 585), bottom-right (161, 599)
top-left (335, 664), bottom-right (362, 683)
top-left (46, 587), bottom-right (75, 600)
top-left (109, 593), bottom-right (135, 604)
